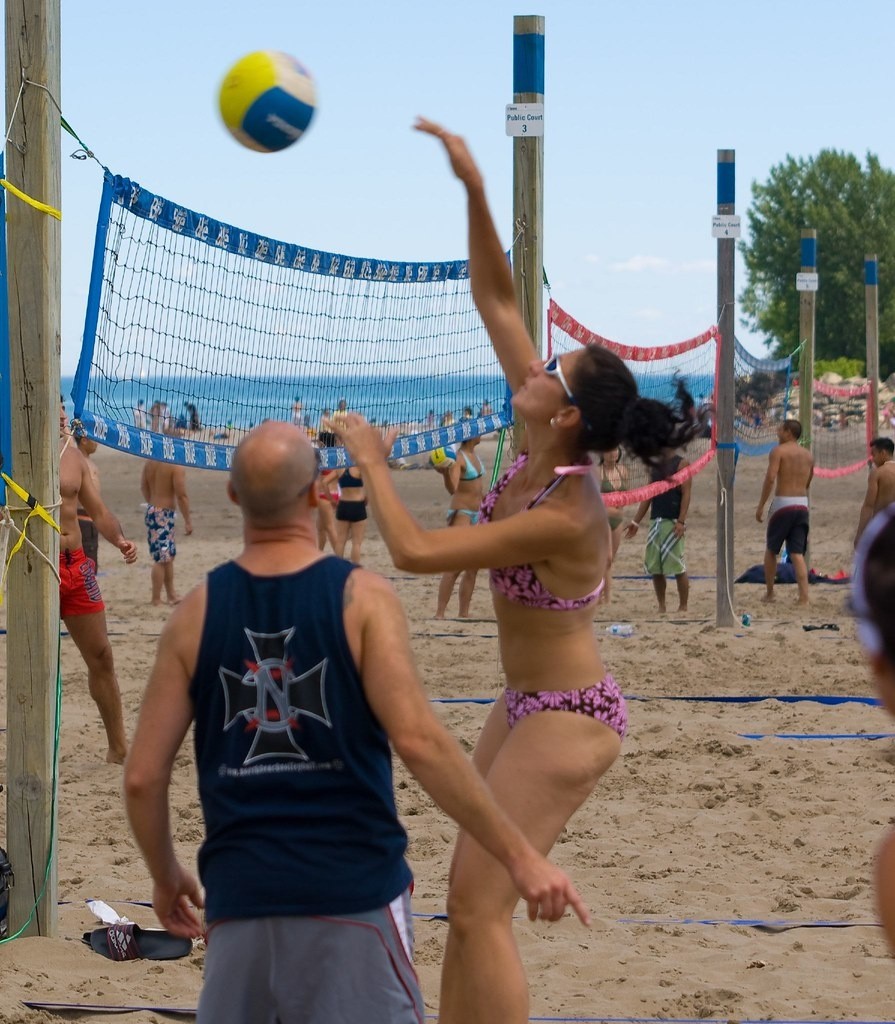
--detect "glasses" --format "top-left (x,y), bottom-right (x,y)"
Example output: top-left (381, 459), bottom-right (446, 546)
top-left (545, 354), bottom-right (579, 406)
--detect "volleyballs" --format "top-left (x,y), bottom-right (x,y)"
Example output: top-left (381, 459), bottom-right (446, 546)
top-left (429, 443), bottom-right (456, 470)
top-left (218, 49), bottom-right (318, 153)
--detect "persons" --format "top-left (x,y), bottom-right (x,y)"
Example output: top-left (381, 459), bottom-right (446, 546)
top-left (854, 436), bottom-right (892, 955)
top-left (757, 421), bottom-right (816, 605)
top-left (324, 118), bottom-right (627, 1021)
top-left (125, 421), bottom-right (592, 1023)
top-left (59, 394), bottom-right (716, 765)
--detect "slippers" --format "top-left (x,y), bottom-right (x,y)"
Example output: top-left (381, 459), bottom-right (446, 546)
top-left (803, 623), bottom-right (839, 630)
top-left (90, 923), bottom-right (192, 961)
